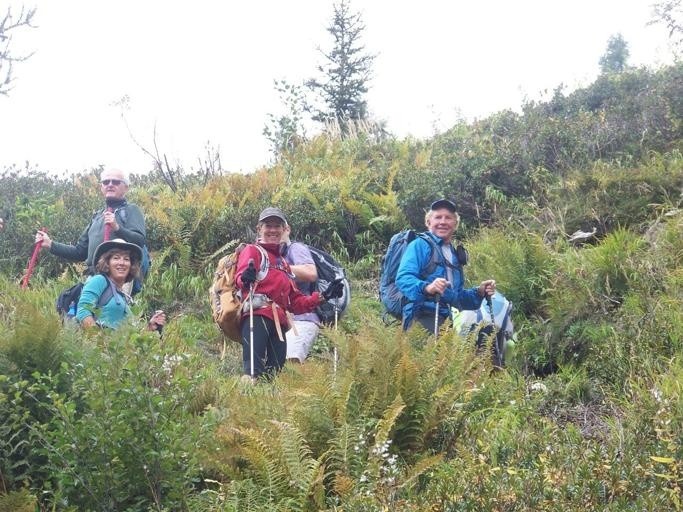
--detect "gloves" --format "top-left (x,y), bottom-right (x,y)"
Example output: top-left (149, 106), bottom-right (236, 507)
top-left (322, 278), bottom-right (343, 300)
top-left (240, 263), bottom-right (257, 287)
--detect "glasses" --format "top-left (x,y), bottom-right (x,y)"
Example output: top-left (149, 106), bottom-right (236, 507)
top-left (100, 179), bottom-right (123, 185)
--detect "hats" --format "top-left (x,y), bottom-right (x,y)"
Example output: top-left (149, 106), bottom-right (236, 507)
top-left (257, 206), bottom-right (287, 225)
top-left (430, 197), bottom-right (455, 211)
top-left (92, 237), bottom-right (144, 277)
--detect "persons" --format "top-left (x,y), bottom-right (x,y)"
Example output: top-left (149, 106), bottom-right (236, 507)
top-left (234, 206), bottom-right (345, 383)
top-left (394, 197), bottom-right (497, 343)
top-left (73, 237), bottom-right (167, 346)
top-left (277, 216), bottom-right (322, 369)
top-left (34, 163), bottom-right (147, 303)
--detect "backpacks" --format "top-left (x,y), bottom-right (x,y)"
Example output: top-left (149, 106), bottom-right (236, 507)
top-left (376, 227), bottom-right (461, 326)
top-left (55, 273), bottom-right (120, 324)
top-left (280, 241), bottom-right (348, 323)
top-left (468, 308), bottom-right (509, 372)
top-left (119, 206), bottom-right (150, 294)
top-left (209, 241), bottom-right (298, 343)
